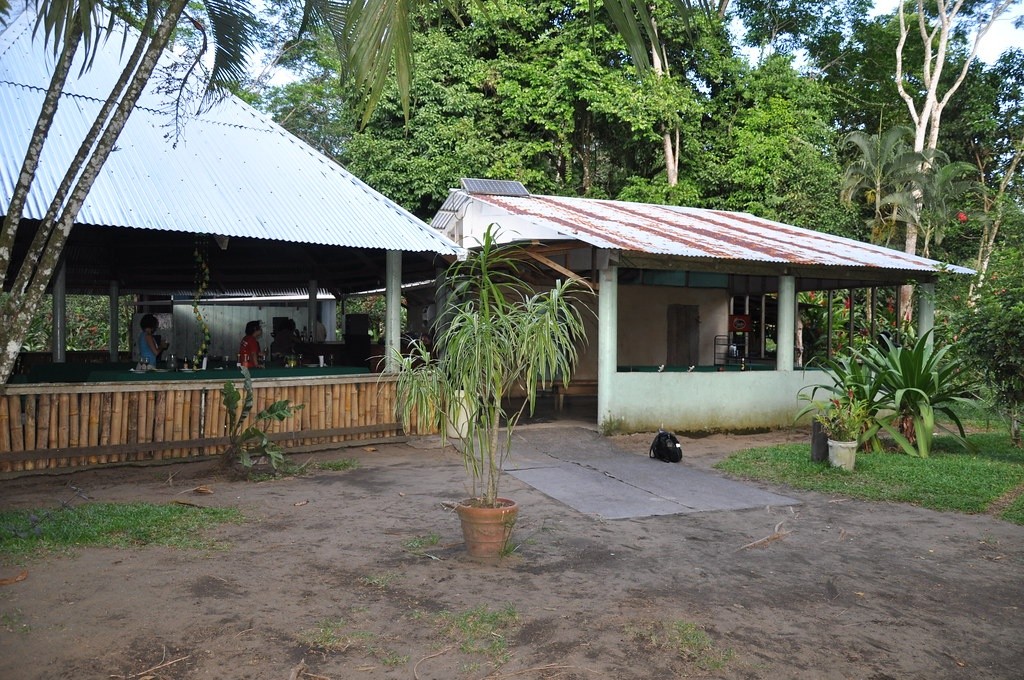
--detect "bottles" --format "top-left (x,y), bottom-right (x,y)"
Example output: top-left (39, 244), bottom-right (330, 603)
top-left (298, 354), bottom-right (303, 369)
top-left (237, 354), bottom-right (241, 371)
top-left (242, 354), bottom-right (248, 368)
top-left (300, 332), bottom-right (304, 341)
top-left (183, 356), bottom-right (189, 369)
top-left (309, 331), bottom-right (313, 343)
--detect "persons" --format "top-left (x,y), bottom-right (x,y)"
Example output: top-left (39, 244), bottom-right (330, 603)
top-left (276, 320), bottom-right (298, 346)
top-left (796, 306), bottom-right (805, 366)
top-left (139, 314), bottom-right (167, 368)
top-left (316, 315), bottom-right (327, 343)
top-left (238, 320), bottom-right (264, 368)
top-left (879, 331), bottom-right (891, 354)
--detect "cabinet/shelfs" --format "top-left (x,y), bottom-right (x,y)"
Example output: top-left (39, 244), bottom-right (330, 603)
top-left (714, 334), bottom-right (751, 371)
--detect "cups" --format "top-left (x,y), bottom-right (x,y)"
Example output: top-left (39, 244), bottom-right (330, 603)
top-left (318, 355), bottom-right (324, 367)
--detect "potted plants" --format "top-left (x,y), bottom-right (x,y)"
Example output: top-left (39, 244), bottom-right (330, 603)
top-left (360, 224), bottom-right (600, 563)
top-left (810, 388), bottom-right (879, 471)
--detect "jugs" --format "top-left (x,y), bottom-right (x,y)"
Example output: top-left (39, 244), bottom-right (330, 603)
top-left (284, 354), bottom-right (299, 369)
top-left (165, 352), bottom-right (179, 373)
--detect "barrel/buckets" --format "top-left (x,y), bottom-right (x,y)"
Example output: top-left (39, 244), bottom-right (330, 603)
top-left (447, 390), bottom-right (478, 438)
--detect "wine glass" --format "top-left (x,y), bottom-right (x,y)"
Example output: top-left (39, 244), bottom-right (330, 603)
top-left (140, 356), bottom-right (150, 371)
